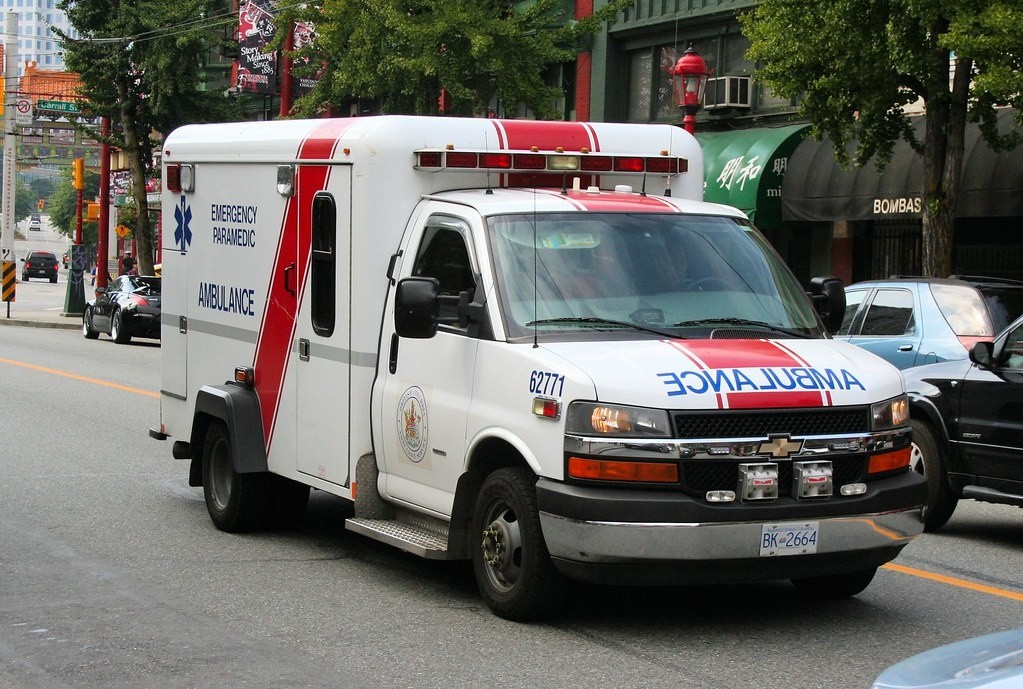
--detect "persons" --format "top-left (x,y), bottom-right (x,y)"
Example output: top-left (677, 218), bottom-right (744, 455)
top-left (644, 243), bottom-right (707, 295)
top-left (121, 251), bottom-right (135, 275)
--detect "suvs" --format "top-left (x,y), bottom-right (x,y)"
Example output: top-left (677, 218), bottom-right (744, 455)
top-left (898, 315), bottom-right (1023, 533)
top-left (29, 221), bottom-right (41, 230)
top-left (20, 250), bottom-right (59, 283)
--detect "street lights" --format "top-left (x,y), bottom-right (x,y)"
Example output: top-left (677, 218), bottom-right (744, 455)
top-left (669, 41), bottom-right (715, 136)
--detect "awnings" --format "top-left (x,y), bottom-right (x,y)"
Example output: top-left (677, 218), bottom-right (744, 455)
top-left (693, 123), bottom-right (813, 229)
top-left (779, 106), bottom-right (1023, 220)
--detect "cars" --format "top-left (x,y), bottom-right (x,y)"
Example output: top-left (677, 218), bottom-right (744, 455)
top-left (82, 275), bottom-right (161, 344)
top-left (840, 273), bottom-right (1023, 372)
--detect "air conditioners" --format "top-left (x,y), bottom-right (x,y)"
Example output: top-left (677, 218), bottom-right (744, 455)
top-left (703, 74), bottom-right (754, 111)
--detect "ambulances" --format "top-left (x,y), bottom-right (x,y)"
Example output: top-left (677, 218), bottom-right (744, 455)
top-left (157, 116), bottom-right (927, 621)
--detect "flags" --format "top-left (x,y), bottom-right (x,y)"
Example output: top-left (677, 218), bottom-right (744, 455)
top-left (292, 3), bottom-right (333, 92)
top-left (236, 4), bottom-right (281, 96)
